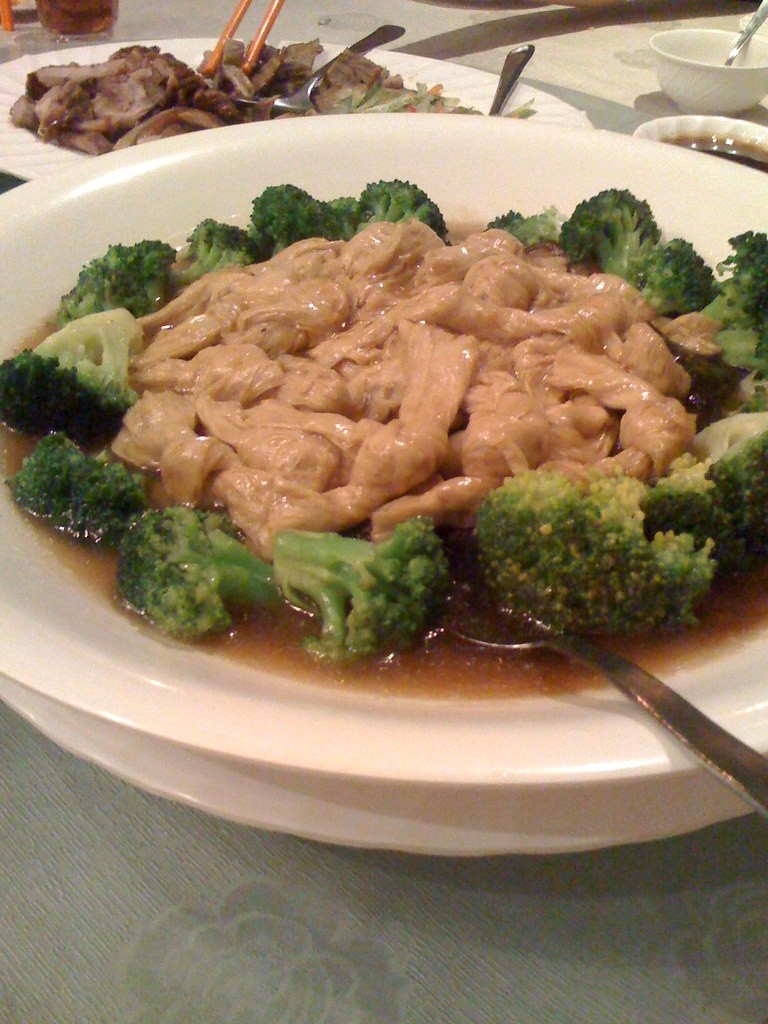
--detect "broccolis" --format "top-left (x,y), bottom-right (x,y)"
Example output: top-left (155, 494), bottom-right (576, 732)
top-left (0, 176), bottom-right (768, 663)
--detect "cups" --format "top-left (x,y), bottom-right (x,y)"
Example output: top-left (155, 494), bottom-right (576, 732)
top-left (35, 0), bottom-right (119, 44)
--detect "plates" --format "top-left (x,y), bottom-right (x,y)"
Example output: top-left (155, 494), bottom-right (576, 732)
top-left (0, 38), bottom-right (595, 182)
top-left (0, 675), bottom-right (756, 859)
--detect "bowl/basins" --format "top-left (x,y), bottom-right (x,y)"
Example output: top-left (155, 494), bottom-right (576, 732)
top-left (0, 112), bottom-right (767, 785)
top-left (632, 115), bottom-right (768, 174)
top-left (648, 28), bottom-right (768, 116)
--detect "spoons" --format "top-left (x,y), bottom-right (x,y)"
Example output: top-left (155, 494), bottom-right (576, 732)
top-left (439, 595), bottom-right (768, 822)
top-left (232, 24), bottom-right (407, 116)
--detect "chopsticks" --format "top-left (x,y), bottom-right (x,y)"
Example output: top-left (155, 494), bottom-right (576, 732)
top-left (202, 0), bottom-right (286, 78)
